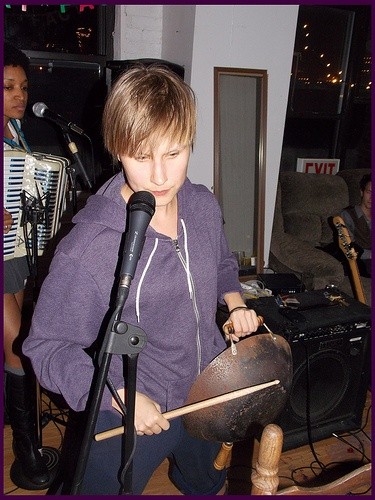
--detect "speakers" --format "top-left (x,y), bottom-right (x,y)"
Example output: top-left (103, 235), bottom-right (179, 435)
top-left (243, 286), bottom-right (371, 452)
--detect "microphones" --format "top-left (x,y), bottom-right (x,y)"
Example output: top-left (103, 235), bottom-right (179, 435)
top-left (32, 102), bottom-right (83, 134)
top-left (19, 190), bottom-right (50, 228)
top-left (116, 191), bottom-right (156, 307)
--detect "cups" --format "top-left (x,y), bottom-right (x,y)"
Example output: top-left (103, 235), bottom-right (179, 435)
top-left (231, 251), bottom-right (245, 266)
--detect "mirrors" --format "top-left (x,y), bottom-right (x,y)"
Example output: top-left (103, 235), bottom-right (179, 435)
top-left (214, 67), bottom-right (268, 282)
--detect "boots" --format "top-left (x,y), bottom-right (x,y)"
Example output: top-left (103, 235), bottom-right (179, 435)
top-left (4, 365), bottom-right (49, 488)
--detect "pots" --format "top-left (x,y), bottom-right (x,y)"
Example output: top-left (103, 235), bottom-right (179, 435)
top-left (182, 315), bottom-right (293, 471)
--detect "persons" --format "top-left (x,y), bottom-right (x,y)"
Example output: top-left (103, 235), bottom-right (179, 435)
top-left (341, 180), bottom-right (373, 279)
top-left (2, 39), bottom-right (75, 422)
top-left (22, 63), bottom-right (260, 497)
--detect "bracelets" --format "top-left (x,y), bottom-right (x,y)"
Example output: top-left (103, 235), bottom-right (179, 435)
top-left (230, 307), bottom-right (247, 315)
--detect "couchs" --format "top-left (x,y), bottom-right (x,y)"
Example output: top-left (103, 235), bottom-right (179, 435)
top-left (270, 169), bottom-right (372, 307)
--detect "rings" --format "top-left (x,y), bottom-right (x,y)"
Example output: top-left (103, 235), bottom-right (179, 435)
top-left (6, 225), bottom-right (8, 229)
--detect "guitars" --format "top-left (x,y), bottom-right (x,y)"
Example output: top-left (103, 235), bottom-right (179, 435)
top-left (333, 215), bottom-right (368, 306)
